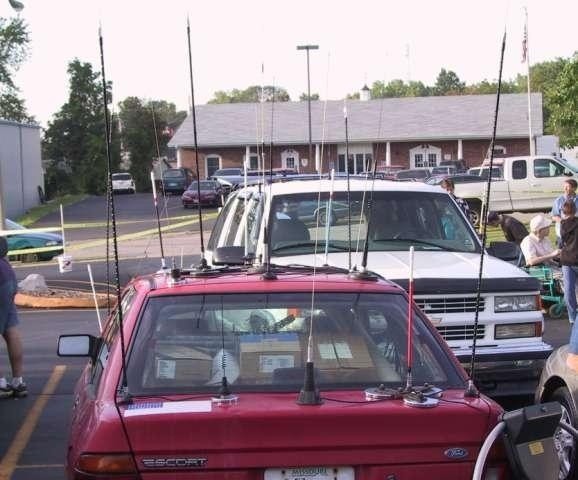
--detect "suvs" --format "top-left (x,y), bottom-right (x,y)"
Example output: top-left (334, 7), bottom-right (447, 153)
top-left (207, 168), bottom-right (553, 399)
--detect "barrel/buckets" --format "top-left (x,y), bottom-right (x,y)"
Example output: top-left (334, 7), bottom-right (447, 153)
top-left (58, 256), bottom-right (72, 273)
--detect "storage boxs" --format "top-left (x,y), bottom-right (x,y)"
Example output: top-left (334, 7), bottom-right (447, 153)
top-left (234, 331), bottom-right (302, 379)
top-left (302, 329), bottom-right (373, 384)
top-left (150, 343), bottom-right (213, 386)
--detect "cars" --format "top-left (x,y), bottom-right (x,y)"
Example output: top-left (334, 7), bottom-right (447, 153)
top-left (53, 260), bottom-right (505, 480)
top-left (0, 217), bottom-right (64, 264)
top-left (526, 335), bottom-right (576, 480)
top-left (109, 171), bottom-right (136, 194)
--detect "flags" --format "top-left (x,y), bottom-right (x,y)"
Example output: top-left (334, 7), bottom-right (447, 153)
top-left (520, 24), bottom-right (527, 65)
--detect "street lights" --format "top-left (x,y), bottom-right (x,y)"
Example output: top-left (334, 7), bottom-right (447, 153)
top-left (293, 42), bottom-right (321, 172)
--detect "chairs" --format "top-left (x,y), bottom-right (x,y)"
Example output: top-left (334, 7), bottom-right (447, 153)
top-left (365, 215), bottom-right (421, 241)
top-left (270, 218), bottom-right (310, 250)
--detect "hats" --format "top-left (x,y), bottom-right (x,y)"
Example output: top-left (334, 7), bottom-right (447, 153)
top-left (530, 214), bottom-right (552, 231)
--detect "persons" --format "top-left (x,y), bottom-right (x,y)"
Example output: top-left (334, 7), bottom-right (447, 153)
top-left (435, 179), bottom-right (470, 240)
top-left (486, 211), bottom-right (530, 243)
top-left (551, 178), bottom-right (578, 249)
top-left (558, 201), bottom-right (578, 323)
top-left (519, 215), bottom-right (565, 293)
top-left (566, 314), bottom-right (578, 371)
top-left (0, 257), bottom-right (28, 399)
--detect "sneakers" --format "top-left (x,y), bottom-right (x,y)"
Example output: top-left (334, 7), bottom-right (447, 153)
top-left (0, 383), bottom-right (14, 399)
top-left (12, 383), bottom-right (28, 398)
